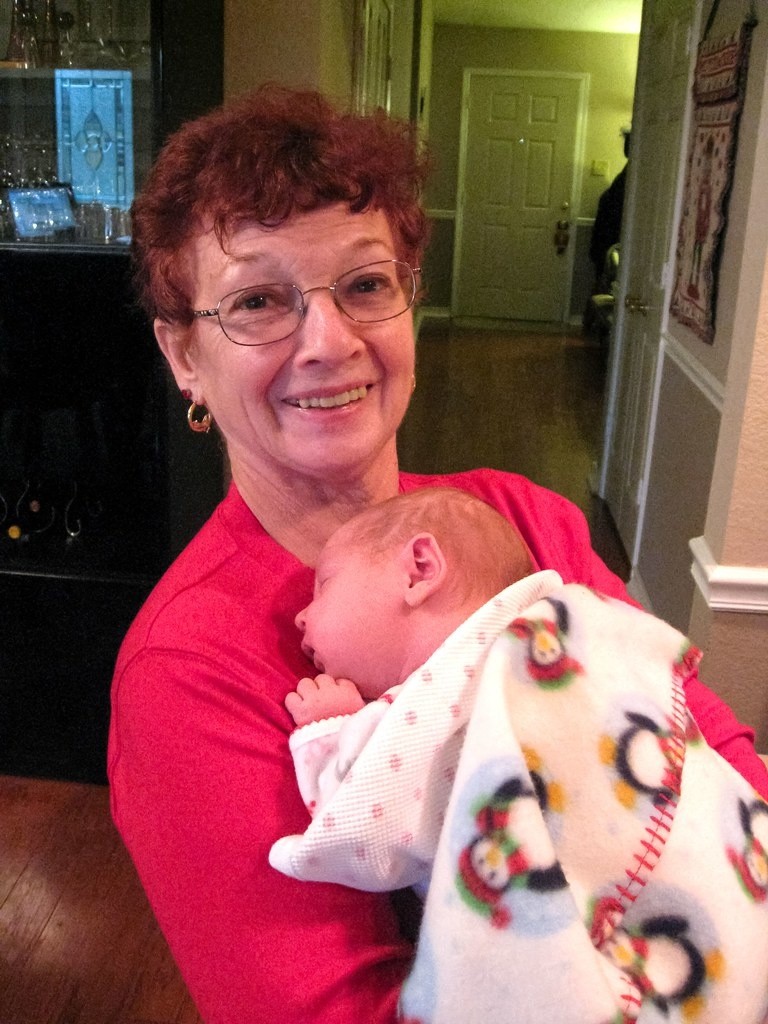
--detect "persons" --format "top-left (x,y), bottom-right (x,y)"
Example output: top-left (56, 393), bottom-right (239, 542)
top-left (106, 86), bottom-right (768, 1024)
top-left (268, 490), bottom-right (768, 1024)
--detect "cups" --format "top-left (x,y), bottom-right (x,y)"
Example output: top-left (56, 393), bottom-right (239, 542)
top-left (0, 0), bottom-right (152, 248)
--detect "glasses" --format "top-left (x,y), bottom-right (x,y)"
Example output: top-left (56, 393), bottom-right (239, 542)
top-left (187, 259), bottom-right (424, 347)
